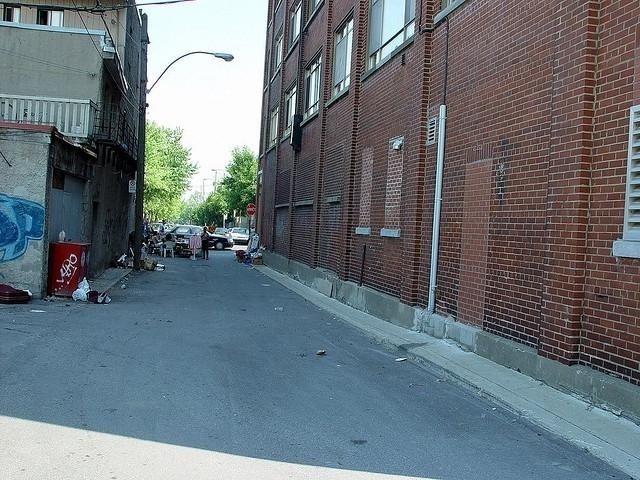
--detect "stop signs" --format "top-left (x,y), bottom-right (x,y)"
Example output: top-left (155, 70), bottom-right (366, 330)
top-left (246, 203), bottom-right (256, 215)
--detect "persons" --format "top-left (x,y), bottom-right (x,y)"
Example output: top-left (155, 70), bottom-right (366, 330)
top-left (142, 218), bottom-right (216, 260)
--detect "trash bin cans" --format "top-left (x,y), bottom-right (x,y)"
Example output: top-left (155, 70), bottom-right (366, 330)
top-left (48, 241), bottom-right (91, 296)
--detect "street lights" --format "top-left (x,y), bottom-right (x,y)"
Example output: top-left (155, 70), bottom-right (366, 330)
top-left (134, 52), bottom-right (234, 269)
top-left (202, 168), bottom-right (217, 193)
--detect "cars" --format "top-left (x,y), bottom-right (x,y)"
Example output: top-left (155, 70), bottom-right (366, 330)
top-left (150, 223), bottom-right (249, 250)
